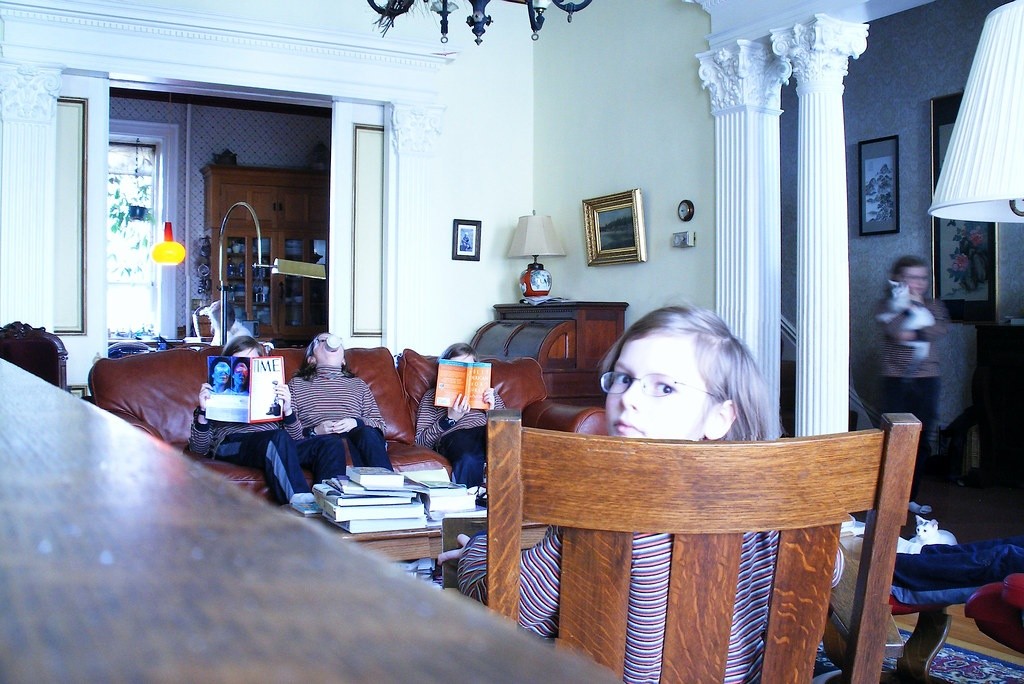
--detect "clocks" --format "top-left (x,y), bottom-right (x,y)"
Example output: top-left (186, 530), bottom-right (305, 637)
top-left (678, 201), bottom-right (694, 220)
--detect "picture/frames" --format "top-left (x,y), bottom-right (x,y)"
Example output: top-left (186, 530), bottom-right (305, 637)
top-left (453, 218), bottom-right (483, 263)
top-left (582, 187), bottom-right (649, 269)
top-left (858, 132), bottom-right (902, 239)
top-left (930, 93), bottom-right (1003, 326)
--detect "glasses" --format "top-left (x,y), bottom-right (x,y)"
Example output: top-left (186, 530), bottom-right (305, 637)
top-left (599, 371), bottom-right (725, 402)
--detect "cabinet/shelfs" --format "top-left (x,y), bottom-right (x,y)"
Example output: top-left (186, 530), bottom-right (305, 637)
top-left (199, 165), bottom-right (334, 341)
top-left (973, 325), bottom-right (1024, 492)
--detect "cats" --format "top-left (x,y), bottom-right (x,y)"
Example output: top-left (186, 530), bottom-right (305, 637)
top-left (875, 278), bottom-right (937, 362)
top-left (908, 514), bottom-right (958, 546)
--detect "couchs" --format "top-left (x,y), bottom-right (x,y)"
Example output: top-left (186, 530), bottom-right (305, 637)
top-left (84, 342), bottom-right (609, 509)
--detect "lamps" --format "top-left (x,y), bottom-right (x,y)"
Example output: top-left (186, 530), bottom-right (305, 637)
top-left (149, 219), bottom-right (187, 265)
top-left (508, 208), bottom-right (566, 297)
top-left (927, 0), bottom-right (1024, 228)
top-left (368, 0), bottom-right (590, 47)
top-left (216, 201), bottom-right (327, 345)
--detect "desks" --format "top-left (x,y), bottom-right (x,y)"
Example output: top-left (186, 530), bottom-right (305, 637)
top-left (442, 515), bottom-right (487, 588)
top-left (471, 300), bottom-right (625, 366)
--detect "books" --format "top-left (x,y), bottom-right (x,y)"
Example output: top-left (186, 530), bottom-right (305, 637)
top-left (204, 355), bottom-right (285, 424)
top-left (434, 358), bottom-right (492, 411)
top-left (290, 467), bottom-right (488, 590)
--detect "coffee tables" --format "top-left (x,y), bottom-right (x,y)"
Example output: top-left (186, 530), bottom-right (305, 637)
top-left (299, 515), bottom-right (553, 591)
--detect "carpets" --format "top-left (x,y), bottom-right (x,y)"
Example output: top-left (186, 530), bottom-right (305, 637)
top-left (808, 623), bottom-right (1024, 682)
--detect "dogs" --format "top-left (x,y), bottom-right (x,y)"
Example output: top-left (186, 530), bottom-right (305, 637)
top-left (199, 301), bottom-right (275, 350)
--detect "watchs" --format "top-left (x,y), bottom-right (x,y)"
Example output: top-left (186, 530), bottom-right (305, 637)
top-left (444, 415), bottom-right (457, 427)
top-left (309, 425), bottom-right (316, 438)
top-left (196, 406), bottom-right (205, 416)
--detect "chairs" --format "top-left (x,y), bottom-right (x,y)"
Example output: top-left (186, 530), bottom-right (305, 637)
top-left (1, 321), bottom-right (73, 393)
top-left (481, 409), bottom-right (927, 683)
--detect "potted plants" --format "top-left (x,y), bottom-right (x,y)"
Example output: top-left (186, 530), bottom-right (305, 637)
top-left (113, 184), bottom-right (162, 278)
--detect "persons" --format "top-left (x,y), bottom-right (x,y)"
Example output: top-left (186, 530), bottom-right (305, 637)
top-left (188, 335), bottom-right (348, 505)
top-left (875, 255), bottom-right (951, 498)
top-left (463, 233), bottom-right (470, 250)
top-left (211, 362), bottom-right (231, 394)
top-left (414, 342), bottom-right (507, 487)
top-left (437, 304), bottom-right (846, 684)
top-left (208, 299), bottom-right (253, 346)
top-left (233, 362), bottom-right (249, 393)
top-left (287, 331), bottom-right (394, 483)
